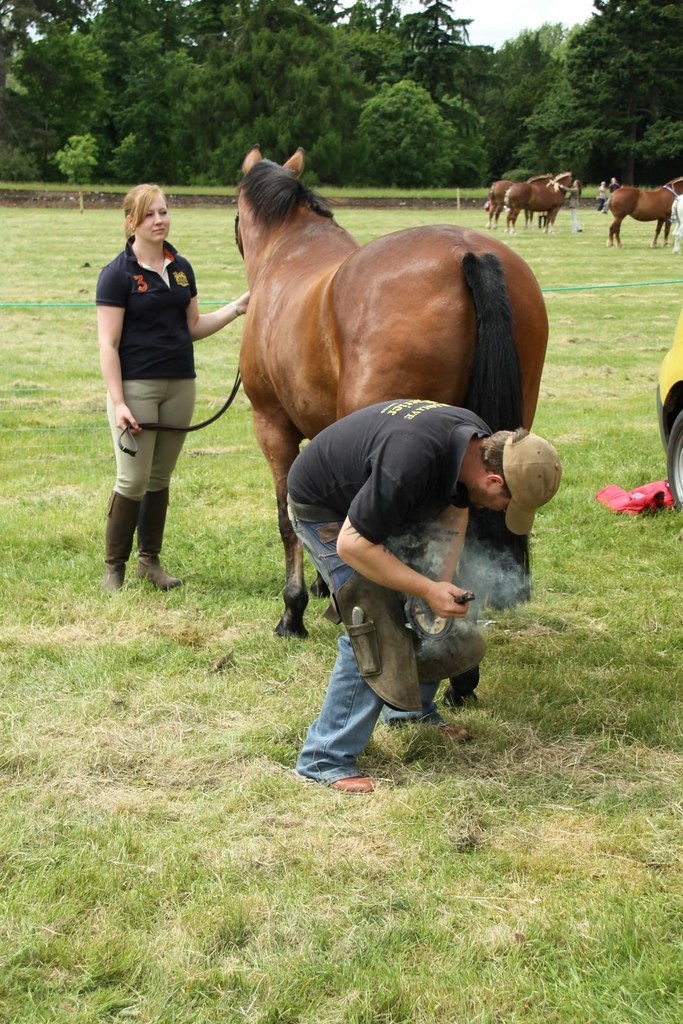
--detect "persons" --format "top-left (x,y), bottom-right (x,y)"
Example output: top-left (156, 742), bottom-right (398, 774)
top-left (96, 184), bottom-right (252, 594)
top-left (598, 181), bottom-right (606, 211)
top-left (560, 179), bottom-right (582, 233)
top-left (288, 398), bottom-right (562, 793)
top-left (602, 177), bottom-right (619, 214)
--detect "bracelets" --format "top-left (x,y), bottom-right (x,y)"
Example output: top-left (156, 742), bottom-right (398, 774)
top-left (232, 303), bottom-right (241, 316)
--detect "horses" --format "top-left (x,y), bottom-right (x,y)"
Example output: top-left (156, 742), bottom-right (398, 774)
top-left (488, 171), bottom-right (573, 232)
top-left (235, 145), bottom-right (551, 706)
top-left (602, 176), bottom-right (683, 249)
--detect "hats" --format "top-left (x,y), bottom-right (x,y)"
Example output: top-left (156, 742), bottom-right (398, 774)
top-left (502, 431), bottom-right (562, 535)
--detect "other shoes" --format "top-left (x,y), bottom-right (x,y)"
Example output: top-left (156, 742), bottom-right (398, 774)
top-left (437, 725), bottom-right (470, 743)
top-left (329, 776), bottom-right (375, 795)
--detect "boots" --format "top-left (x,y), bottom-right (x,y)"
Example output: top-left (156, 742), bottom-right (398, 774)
top-left (137, 487), bottom-right (182, 590)
top-left (100, 490), bottom-right (141, 594)
